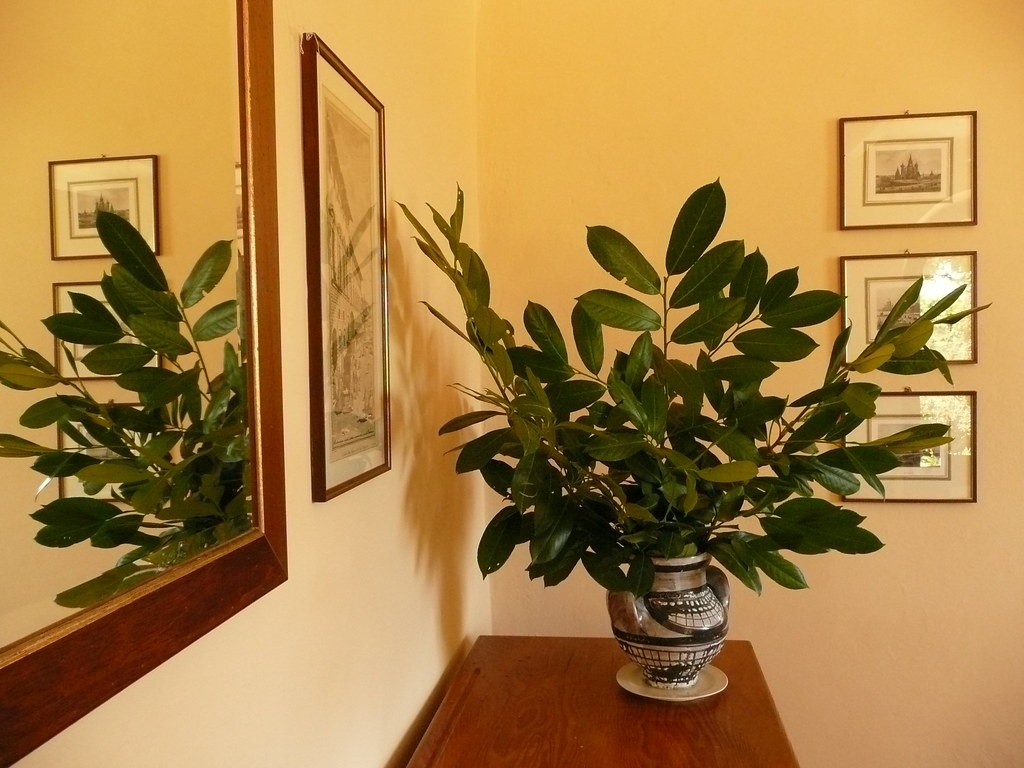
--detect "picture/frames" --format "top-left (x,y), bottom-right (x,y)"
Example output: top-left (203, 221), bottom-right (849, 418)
top-left (841, 390), bottom-right (978, 504)
top-left (53, 280), bottom-right (163, 381)
top-left (48, 154), bottom-right (160, 262)
top-left (300, 31), bottom-right (392, 503)
top-left (58, 401), bottom-right (159, 503)
top-left (838, 110), bottom-right (978, 231)
top-left (839, 251), bottom-right (978, 366)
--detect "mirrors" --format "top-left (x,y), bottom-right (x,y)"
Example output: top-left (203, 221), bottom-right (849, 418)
top-left (0, 0), bottom-right (289, 768)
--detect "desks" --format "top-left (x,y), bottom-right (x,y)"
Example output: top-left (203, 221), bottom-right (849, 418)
top-left (403, 634), bottom-right (800, 768)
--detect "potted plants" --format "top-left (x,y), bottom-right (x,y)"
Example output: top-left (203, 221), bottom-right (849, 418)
top-left (394, 177), bottom-right (992, 690)
